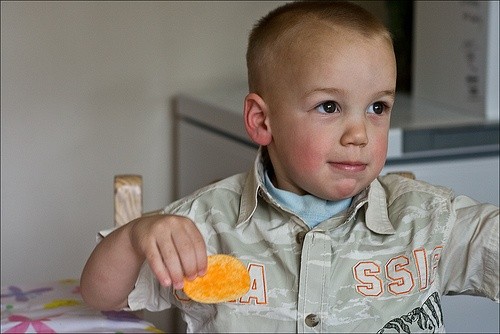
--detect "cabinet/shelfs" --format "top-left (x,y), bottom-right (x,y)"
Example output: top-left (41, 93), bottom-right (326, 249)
top-left (171, 83), bottom-right (500, 333)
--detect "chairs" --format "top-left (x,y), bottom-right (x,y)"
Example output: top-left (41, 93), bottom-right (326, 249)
top-left (113, 170), bottom-right (416, 229)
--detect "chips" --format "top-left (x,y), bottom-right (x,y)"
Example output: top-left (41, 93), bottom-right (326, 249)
top-left (180, 253), bottom-right (251, 304)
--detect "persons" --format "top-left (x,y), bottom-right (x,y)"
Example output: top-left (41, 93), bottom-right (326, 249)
top-left (80, 0), bottom-right (500, 334)
